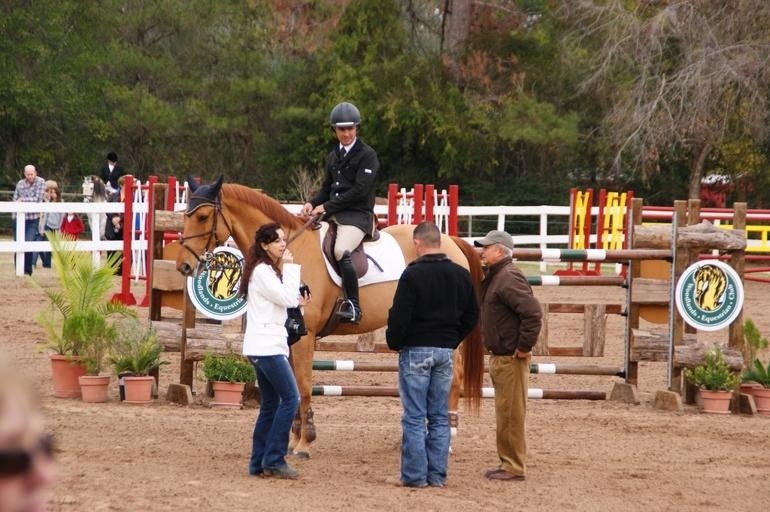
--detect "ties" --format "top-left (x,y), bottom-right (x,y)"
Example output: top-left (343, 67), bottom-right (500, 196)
top-left (339, 148), bottom-right (346, 160)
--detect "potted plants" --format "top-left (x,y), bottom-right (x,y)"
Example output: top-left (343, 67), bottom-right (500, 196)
top-left (690, 352), bottom-right (738, 411)
top-left (737, 319), bottom-right (770, 396)
top-left (26, 233), bottom-right (141, 398)
top-left (743, 358), bottom-right (770, 416)
top-left (116, 323), bottom-right (165, 405)
top-left (204, 352), bottom-right (255, 402)
top-left (64, 314), bottom-right (113, 400)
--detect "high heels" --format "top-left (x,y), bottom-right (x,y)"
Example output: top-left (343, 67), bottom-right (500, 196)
top-left (263, 466), bottom-right (297, 476)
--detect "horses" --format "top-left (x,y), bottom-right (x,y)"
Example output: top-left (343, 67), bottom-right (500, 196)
top-left (175, 173), bottom-right (486, 461)
top-left (82, 174), bottom-right (111, 255)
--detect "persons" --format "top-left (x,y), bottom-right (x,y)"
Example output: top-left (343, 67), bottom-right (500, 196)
top-left (473, 231), bottom-right (542, 480)
top-left (12, 152), bottom-right (147, 276)
top-left (385, 221), bottom-right (482, 486)
top-left (1, 350), bottom-right (63, 512)
top-left (239, 223), bottom-right (312, 479)
top-left (299, 102), bottom-right (381, 321)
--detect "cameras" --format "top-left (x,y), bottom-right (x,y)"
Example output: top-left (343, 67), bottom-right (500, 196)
top-left (284, 317), bottom-right (300, 336)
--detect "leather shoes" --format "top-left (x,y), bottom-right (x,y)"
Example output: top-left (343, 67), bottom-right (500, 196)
top-left (393, 467), bottom-right (525, 488)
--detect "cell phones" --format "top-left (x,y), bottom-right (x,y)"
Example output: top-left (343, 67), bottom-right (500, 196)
top-left (299, 285), bottom-right (310, 298)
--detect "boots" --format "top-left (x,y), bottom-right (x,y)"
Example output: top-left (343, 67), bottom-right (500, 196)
top-left (336, 250), bottom-right (362, 324)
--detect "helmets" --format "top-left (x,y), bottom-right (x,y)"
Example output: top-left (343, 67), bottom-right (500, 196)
top-left (106, 152), bottom-right (118, 163)
top-left (329, 102), bottom-right (361, 128)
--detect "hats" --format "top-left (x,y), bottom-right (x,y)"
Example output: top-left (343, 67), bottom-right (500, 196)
top-left (474, 230), bottom-right (514, 251)
